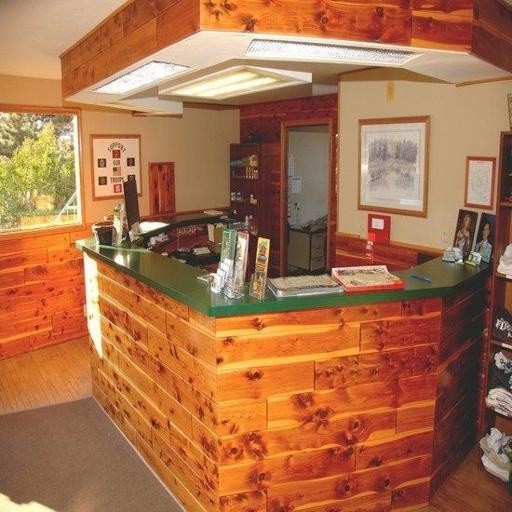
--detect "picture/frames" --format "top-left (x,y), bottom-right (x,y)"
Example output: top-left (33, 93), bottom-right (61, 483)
top-left (464, 155), bottom-right (497, 210)
top-left (88, 133), bottom-right (143, 201)
top-left (358, 114), bottom-right (431, 218)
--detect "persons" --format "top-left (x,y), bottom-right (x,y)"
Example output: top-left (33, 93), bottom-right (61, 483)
top-left (454, 212), bottom-right (473, 259)
top-left (472, 221), bottom-right (493, 263)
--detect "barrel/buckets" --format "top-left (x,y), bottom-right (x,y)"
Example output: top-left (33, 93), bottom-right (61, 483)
top-left (91, 221), bottom-right (113, 245)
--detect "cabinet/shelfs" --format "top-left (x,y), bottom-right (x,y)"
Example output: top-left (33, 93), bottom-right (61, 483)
top-left (476, 130), bottom-right (512, 484)
top-left (286, 224), bottom-right (326, 273)
top-left (229, 143), bottom-right (271, 274)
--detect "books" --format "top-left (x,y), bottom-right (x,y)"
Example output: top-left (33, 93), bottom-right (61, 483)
top-left (265, 273), bottom-right (344, 300)
top-left (331, 264), bottom-right (407, 293)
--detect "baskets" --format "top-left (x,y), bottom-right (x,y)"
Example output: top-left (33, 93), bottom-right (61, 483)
top-left (92, 220), bottom-right (116, 246)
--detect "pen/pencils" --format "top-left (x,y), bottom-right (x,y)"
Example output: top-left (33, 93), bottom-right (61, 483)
top-left (409, 274), bottom-right (432, 283)
